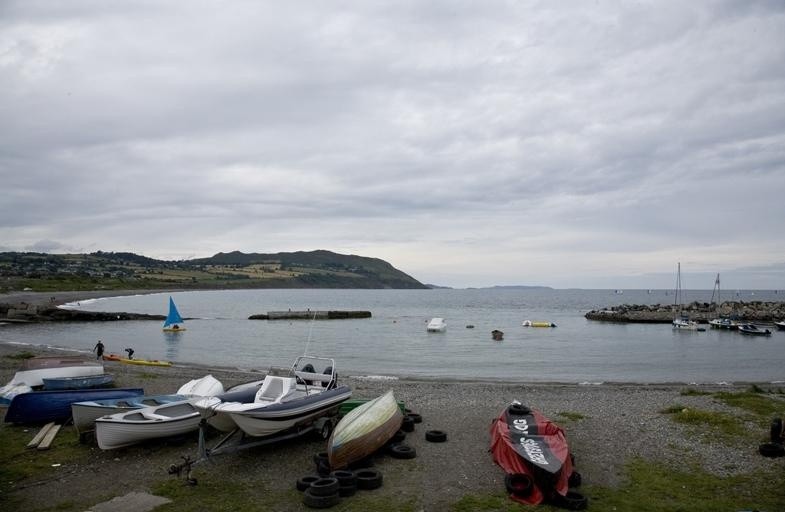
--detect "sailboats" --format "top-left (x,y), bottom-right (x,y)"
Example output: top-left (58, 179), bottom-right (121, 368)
top-left (162, 295), bottom-right (186, 332)
top-left (708, 273), bottom-right (755, 326)
top-left (672, 262), bottom-right (705, 331)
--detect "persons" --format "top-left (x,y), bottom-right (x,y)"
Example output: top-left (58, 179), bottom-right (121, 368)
top-left (124, 347), bottom-right (134, 359)
top-left (93, 339), bottom-right (105, 360)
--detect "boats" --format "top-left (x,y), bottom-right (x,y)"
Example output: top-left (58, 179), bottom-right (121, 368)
top-left (42, 376), bottom-right (113, 389)
top-left (71, 376), bottom-right (265, 450)
top-left (773, 320), bottom-right (785, 328)
top-left (329, 388), bottom-right (404, 471)
top-left (737, 324), bottom-right (772, 335)
top-left (195, 323), bottom-right (352, 438)
top-left (427, 318), bottom-right (447, 331)
top-left (523, 320), bottom-right (531, 326)
top-left (497, 400), bottom-right (568, 474)
top-left (532, 322), bottom-right (557, 327)
top-left (4, 390), bottom-right (144, 424)
